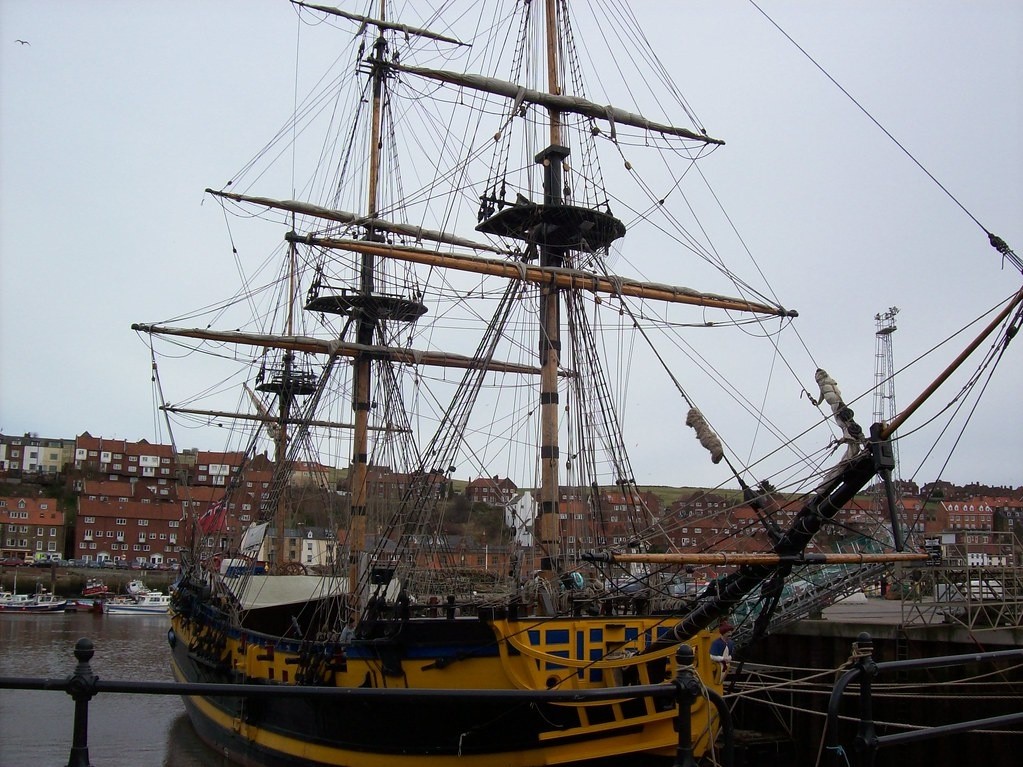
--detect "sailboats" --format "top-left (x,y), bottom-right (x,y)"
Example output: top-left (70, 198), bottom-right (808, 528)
top-left (0, 1), bottom-right (1023, 767)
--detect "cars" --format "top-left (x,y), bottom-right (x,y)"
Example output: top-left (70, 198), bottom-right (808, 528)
top-left (0, 557), bottom-right (23, 567)
top-left (130, 562), bottom-right (141, 570)
top-left (101, 559), bottom-right (114, 569)
top-left (30, 558), bottom-right (58, 569)
top-left (116, 560), bottom-right (128, 570)
